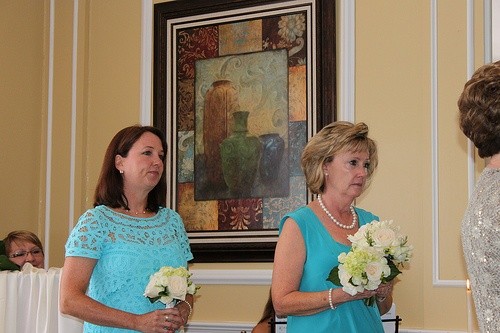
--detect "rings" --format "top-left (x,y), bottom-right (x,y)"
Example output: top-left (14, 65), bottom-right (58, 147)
top-left (166, 314), bottom-right (168, 321)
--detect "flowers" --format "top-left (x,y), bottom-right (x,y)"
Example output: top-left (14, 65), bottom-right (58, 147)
top-left (144, 265), bottom-right (202, 308)
top-left (326, 220), bottom-right (412, 307)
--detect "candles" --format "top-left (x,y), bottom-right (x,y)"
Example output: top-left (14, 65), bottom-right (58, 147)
top-left (466, 279), bottom-right (472, 333)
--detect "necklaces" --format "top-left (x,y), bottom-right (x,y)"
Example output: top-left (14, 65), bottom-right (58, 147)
top-left (126, 208), bottom-right (149, 214)
top-left (318, 194), bottom-right (357, 229)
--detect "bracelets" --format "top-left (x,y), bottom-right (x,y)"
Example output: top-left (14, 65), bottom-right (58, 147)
top-left (177, 300), bottom-right (192, 318)
top-left (328, 288), bottom-right (336, 310)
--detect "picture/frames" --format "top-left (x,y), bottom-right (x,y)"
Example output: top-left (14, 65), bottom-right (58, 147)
top-left (154, 0), bottom-right (336, 262)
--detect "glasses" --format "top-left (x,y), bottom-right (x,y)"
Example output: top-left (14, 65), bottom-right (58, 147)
top-left (9, 248), bottom-right (40, 257)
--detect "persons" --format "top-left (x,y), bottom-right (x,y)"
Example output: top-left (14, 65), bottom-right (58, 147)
top-left (60, 125), bottom-right (194, 333)
top-left (272, 121), bottom-right (393, 333)
top-left (3, 230), bottom-right (44, 269)
top-left (458, 60), bottom-right (500, 333)
